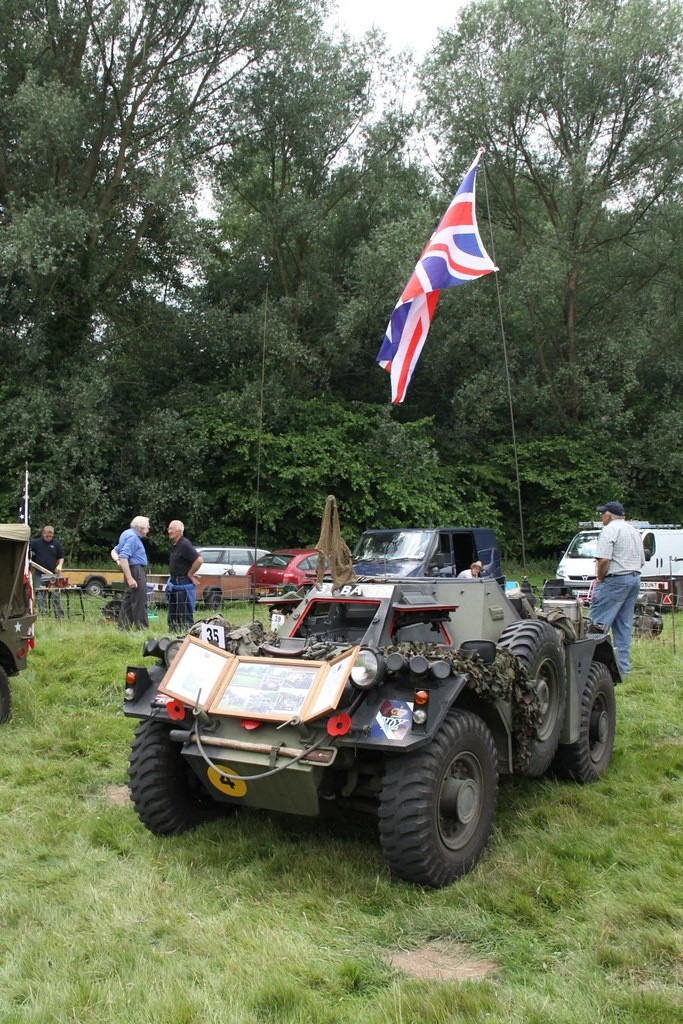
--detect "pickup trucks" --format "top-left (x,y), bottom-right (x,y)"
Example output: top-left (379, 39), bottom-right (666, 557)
top-left (145, 545), bottom-right (287, 610)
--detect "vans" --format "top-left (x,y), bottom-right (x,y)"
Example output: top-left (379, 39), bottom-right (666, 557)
top-left (556, 521), bottom-right (683, 613)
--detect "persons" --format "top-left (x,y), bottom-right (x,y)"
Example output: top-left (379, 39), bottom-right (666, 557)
top-left (29, 525), bottom-right (66, 620)
top-left (110, 516), bottom-right (151, 631)
top-left (163, 520), bottom-right (203, 633)
top-left (585, 502), bottom-right (645, 675)
top-left (455, 561), bottom-right (483, 580)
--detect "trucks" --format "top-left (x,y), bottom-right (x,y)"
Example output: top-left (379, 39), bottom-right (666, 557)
top-left (58, 570), bottom-right (124, 595)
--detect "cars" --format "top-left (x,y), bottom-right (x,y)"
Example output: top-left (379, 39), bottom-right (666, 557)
top-left (246, 547), bottom-right (332, 597)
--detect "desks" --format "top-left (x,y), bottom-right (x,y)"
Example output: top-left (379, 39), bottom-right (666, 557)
top-left (35, 586), bottom-right (86, 622)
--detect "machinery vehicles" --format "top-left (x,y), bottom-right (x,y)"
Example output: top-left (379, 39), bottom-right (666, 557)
top-left (122, 573), bottom-right (624, 892)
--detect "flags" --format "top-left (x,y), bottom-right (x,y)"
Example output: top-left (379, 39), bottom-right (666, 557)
top-left (374, 148), bottom-right (501, 405)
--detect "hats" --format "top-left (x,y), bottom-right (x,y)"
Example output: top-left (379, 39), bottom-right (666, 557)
top-left (597, 502), bottom-right (626, 516)
top-left (470, 561), bottom-right (482, 569)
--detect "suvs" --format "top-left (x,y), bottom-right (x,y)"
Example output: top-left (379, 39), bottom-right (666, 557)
top-left (321, 529), bottom-right (505, 591)
top-left (0, 523), bottom-right (33, 722)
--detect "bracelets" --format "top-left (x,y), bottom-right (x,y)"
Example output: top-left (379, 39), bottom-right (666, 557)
top-left (115, 559), bottom-right (120, 562)
